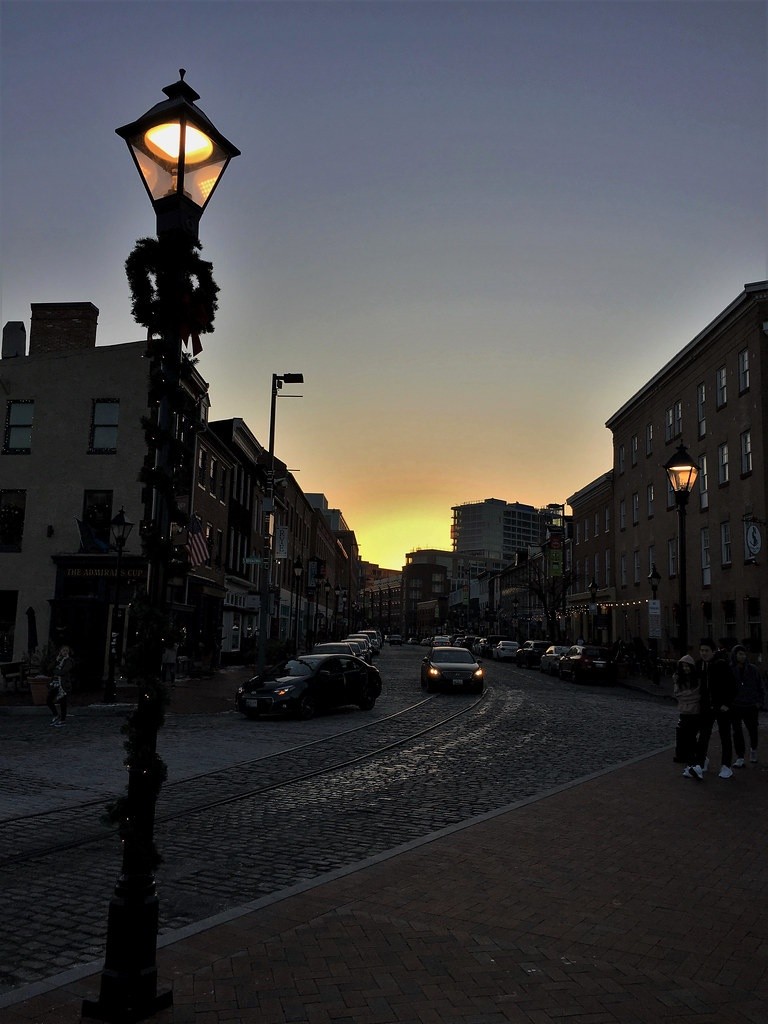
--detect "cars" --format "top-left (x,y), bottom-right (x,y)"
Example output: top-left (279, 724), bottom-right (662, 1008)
top-left (407, 637), bottom-right (419, 645)
top-left (384, 635), bottom-right (391, 643)
top-left (232, 653), bottom-right (383, 720)
top-left (537, 646), bottom-right (571, 676)
top-left (559, 644), bottom-right (616, 684)
top-left (492, 641), bottom-right (522, 662)
top-left (420, 634), bottom-right (512, 658)
top-left (312, 630), bottom-right (383, 664)
top-left (420, 646), bottom-right (484, 694)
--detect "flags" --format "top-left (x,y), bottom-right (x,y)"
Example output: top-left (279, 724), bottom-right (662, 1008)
top-left (181, 514), bottom-right (211, 566)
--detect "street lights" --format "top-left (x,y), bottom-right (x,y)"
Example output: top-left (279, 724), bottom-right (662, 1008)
top-left (661, 439), bottom-right (702, 655)
top-left (586, 580), bottom-right (600, 644)
top-left (108, 504), bottom-right (134, 701)
top-left (511, 595), bottom-right (519, 640)
top-left (324, 577), bottom-right (331, 639)
top-left (335, 583), bottom-right (341, 640)
top-left (260, 372), bottom-right (305, 661)
top-left (293, 553), bottom-right (304, 654)
top-left (341, 590), bottom-right (349, 636)
top-left (352, 600), bottom-right (356, 632)
top-left (69, 64), bottom-right (246, 1024)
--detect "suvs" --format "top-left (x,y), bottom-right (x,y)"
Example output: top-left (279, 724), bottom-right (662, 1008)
top-left (515, 640), bottom-right (554, 670)
top-left (389, 634), bottom-right (404, 646)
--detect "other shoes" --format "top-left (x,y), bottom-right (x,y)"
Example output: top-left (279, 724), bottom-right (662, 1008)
top-left (689, 765), bottom-right (703, 779)
top-left (733, 758), bottom-right (746, 768)
top-left (682, 766), bottom-right (693, 778)
top-left (55, 720), bottom-right (67, 727)
top-left (701, 757), bottom-right (710, 771)
top-left (49, 714), bottom-right (60, 726)
top-left (718, 765), bottom-right (733, 778)
top-left (749, 747), bottom-right (758, 762)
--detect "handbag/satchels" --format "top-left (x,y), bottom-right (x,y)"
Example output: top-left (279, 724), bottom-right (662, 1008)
top-left (57, 677), bottom-right (67, 700)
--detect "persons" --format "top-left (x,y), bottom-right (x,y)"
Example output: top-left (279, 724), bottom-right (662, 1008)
top-left (46, 645), bottom-right (71, 728)
top-left (730, 645), bottom-right (759, 767)
top-left (672, 654), bottom-right (702, 764)
top-left (690, 638), bottom-right (734, 778)
top-left (161, 642), bottom-right (178, 690)
